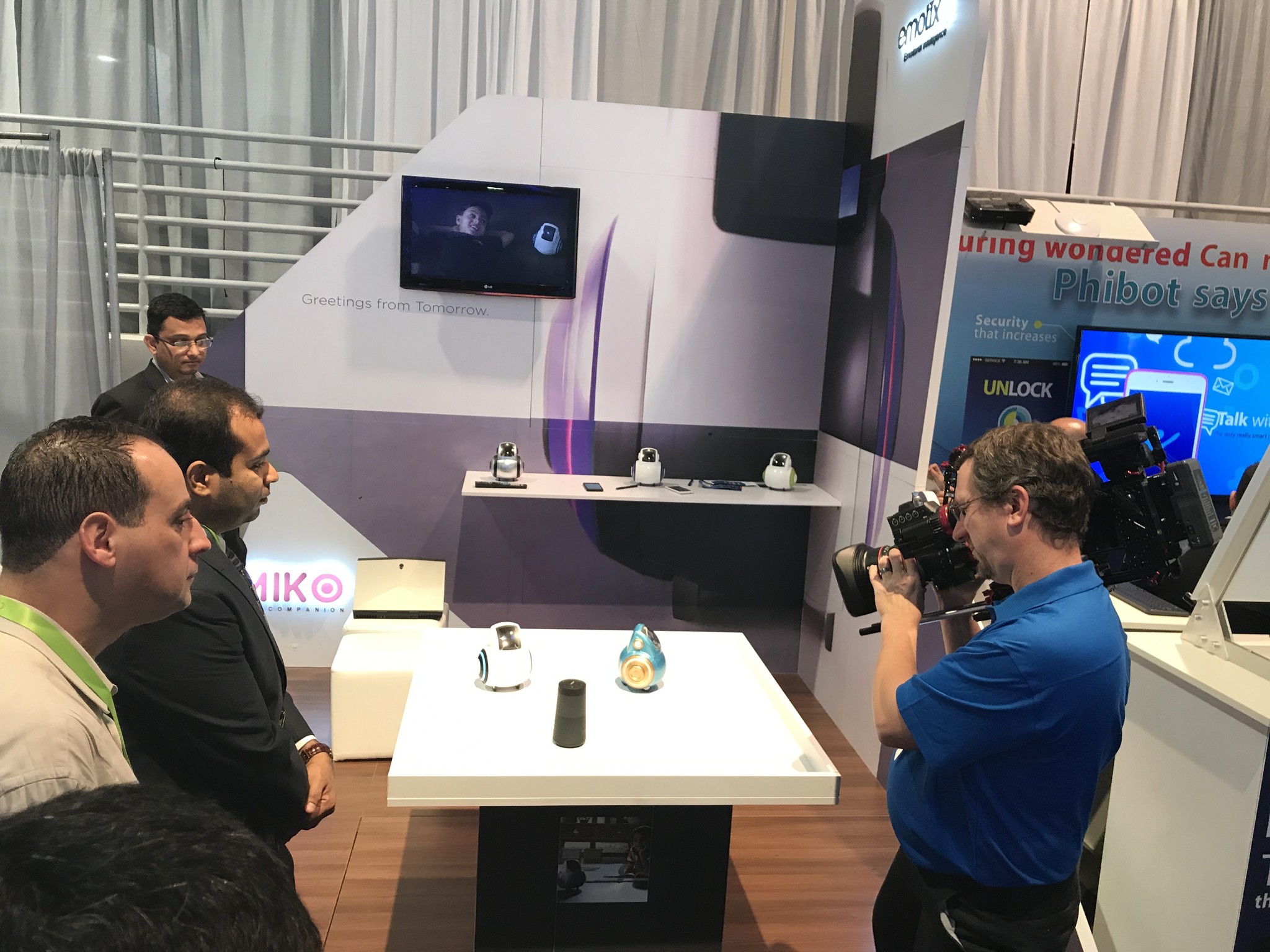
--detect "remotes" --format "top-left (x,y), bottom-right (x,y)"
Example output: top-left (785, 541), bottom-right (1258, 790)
top-left (475, 482), bottom-right (527, 489)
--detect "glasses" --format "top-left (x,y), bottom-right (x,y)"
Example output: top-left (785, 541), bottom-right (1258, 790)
top-left (153, 335), bottom-right (214, 350)
top-left (950, 492), bottom-right (1006, 522)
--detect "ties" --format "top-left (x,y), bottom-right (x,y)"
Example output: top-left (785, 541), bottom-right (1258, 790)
top-left (225, 543), bottom-right (266, 629)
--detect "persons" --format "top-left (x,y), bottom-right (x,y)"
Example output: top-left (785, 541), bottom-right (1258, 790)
top-left (0, 779), bottom-right (324, 952)
top-left (92, 377), bottom-right (338, 846)
top-left (425, 200), bottom-right (514, 248)
top-left (928, 416), bottom-right (1088, 506)
top-left (90, 292), bottom-right (214, 423)
top-left (871, 420), bottom-right (1129, 952)
top-left (1169, 460), bottom-right (1270, 636)
top-left (0, 416), bottom-right (211, 818)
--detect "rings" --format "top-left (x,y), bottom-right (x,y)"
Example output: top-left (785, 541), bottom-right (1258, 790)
top-left (879, 567), bottom-right (892, 575)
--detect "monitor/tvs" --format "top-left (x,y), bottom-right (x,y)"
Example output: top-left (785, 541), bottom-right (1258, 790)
top-left (399, 176), bottom-right (580, 299)
top-left (1065, 324), bottom-right (1270, 501)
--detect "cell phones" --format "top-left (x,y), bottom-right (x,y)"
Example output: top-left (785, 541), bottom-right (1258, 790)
top-left (583, 483), bottom-right (603, 491)
top-left (1123, 369), bottom-right (1207, 477)
top-left (665, 485), bottom-right (693, 494)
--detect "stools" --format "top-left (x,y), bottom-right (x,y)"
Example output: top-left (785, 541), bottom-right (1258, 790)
top-left (331, 602), bottom-right (449, 761)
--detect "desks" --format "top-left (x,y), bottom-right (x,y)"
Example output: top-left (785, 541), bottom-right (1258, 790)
top-left (387, 628), bottom-right (841, 952)
top-left (462, 471), bottom-right (841, 507)
